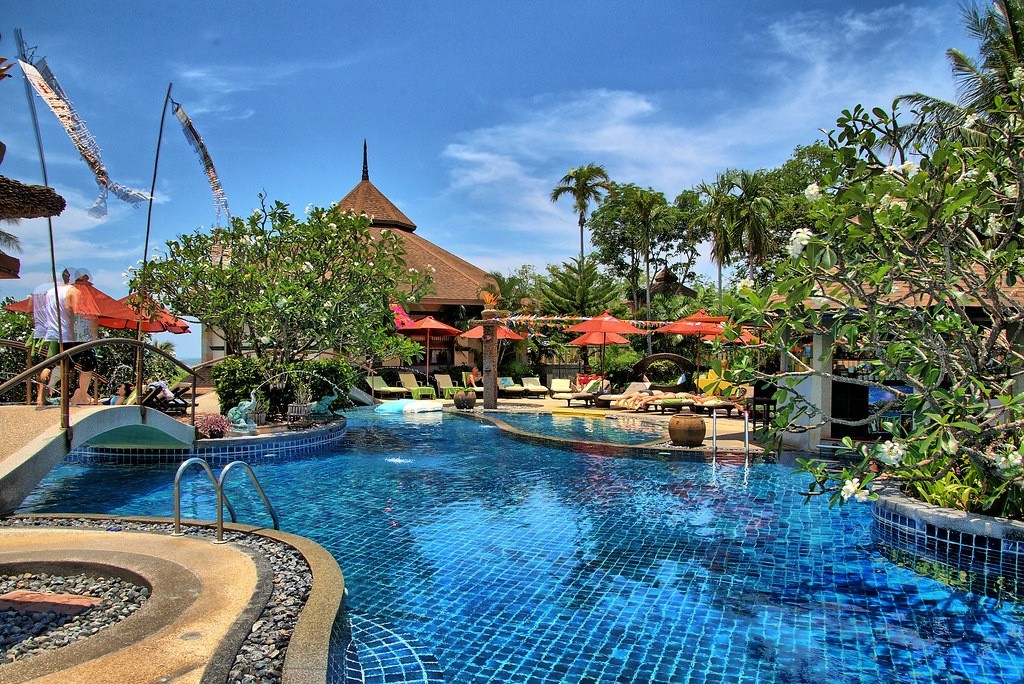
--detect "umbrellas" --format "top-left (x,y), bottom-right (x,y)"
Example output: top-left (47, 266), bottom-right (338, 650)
top-left (4, 273), bottom-right (149, 329)
top-left (460, 323), bottom-right (524, 341)
top-left (566, 310), bottom-right (647, 393)
top-left (655, 306), bottom-right (765, 396)
top-left (96, 289), bottom-right (192, 384)
top-left (396, 316), bottom-right (462, 386)
top-left (570, 331), bottom-right (631, 376)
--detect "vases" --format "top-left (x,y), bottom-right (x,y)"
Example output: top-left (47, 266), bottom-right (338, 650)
top-left (209, 429), bottom-right (224, 439)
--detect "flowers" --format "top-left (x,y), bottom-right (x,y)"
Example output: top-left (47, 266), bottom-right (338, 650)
top-left (196, 415), bottom-right (231, 433)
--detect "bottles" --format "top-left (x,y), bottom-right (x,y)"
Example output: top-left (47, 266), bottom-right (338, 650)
top-left (785, 344), bottom-right (908, 381)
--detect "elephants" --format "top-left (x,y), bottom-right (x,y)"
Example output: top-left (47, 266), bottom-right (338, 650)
top-left (228, 389), bottom-right (256, 424)
top-left (310, 387), bottom-right (338, 417)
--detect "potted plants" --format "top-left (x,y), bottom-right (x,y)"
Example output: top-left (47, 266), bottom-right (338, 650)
top-left (287, 378), bottom-right (318, 431)
top-left (593, 385), bottom-right (611, 408)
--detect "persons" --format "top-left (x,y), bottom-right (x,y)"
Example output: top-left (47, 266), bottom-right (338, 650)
top-left (571, 371), bottom-right (602, 392)
top-left (107, 379), bottom-right (137, 405)
top-left (616, 388), bottom-right (746, 412)
top-left (467, 364), bottom-right (486, 388)
top-left (35, 268), bottom-right (96, 409)
top-left (534, 354), bottom-right (556, 399)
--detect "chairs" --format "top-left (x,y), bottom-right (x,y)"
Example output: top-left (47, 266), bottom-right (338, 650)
top-left (598, 382), bottom-right (652, 401)
top-left (125, 382), bottom-right (199, 417)
top-left (497, 377), bottom-right (525, 399)
top-left (462, 371), bottom-right (484, 392)
top-left (575, 379), bottom-right (609, 409)
top-left (434, 374), bottom-right (465, 400)
top-left (365, 376), bottom-right (409, 400)
top-left (521, 377), bottom-right (548, 399)
top-left (647, 387), bottom-right (755, 417)
top-left (549, 379), bottom-right (579, 407)
top-left (399, 373), bottom-right (436, 400)
